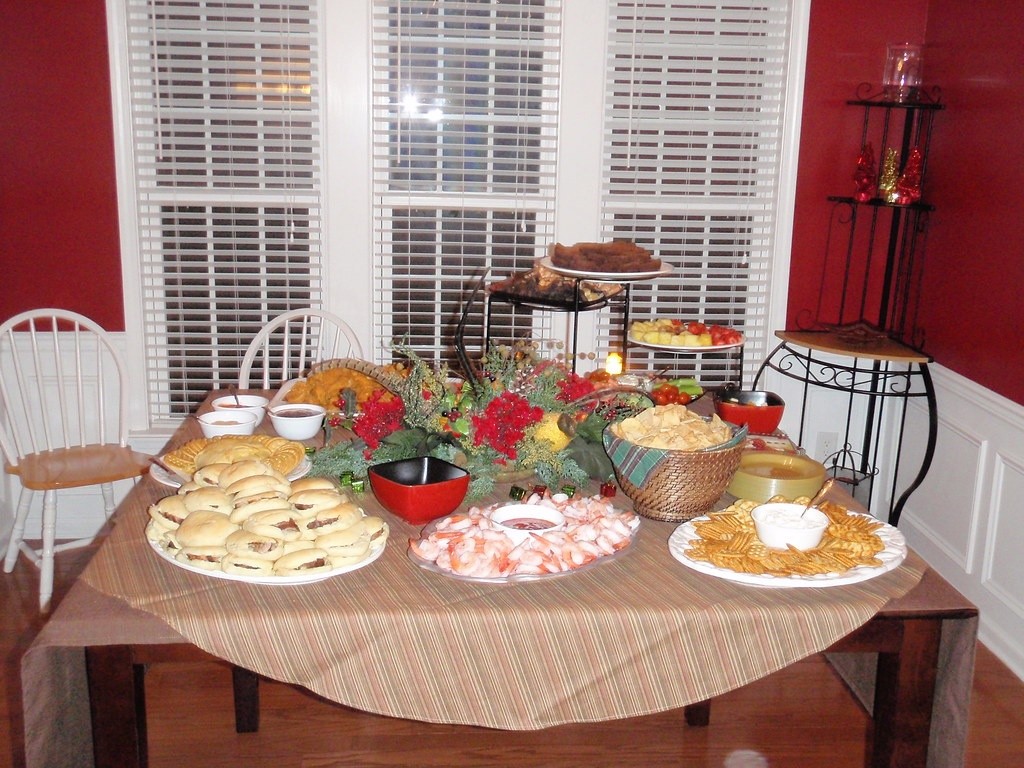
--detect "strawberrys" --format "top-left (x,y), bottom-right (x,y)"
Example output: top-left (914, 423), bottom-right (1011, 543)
top-left (672, 318), bottom-right (742, 345)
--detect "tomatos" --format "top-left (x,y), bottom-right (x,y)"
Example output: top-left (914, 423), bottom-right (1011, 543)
top-left (650, 383), bottom-right (691, 405)
top-left (590, 370), bottom-right (610, 380)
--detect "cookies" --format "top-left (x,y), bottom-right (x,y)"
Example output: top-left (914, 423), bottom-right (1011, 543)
top-left (165, 434), bottom-right (306, 475)
top-left (684, 495), bottom-right (885, 577)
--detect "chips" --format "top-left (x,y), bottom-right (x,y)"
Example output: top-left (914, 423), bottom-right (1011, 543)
top-left (609, 403), bottom-right (732, 449)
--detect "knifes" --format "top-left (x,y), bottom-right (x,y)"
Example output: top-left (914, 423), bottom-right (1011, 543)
top-left (149, 458), bottom-right (185, 484)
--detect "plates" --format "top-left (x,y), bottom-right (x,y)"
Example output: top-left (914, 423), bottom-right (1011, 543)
top-left (726, 453), bottom-right (826, 502)
top-left (408, 502), bottom-right (639, 582)
top-left (150, 453), bottom-right (313, 490)
top-left (653, 382), bottom-right (706, 409)
top-left (491, 288), bottom-right (624, 307)
top-left (148, 514), bottom-right (385, 583)
top-left (540, 256), bottom-right (674, 280)
top-left (667, 509), bottom-right (907, 588)
top-left (628, 332), bottom-right (745, 352)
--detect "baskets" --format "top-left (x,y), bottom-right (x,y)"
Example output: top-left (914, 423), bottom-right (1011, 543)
top-left (605, 422), bottom-right (746, 523)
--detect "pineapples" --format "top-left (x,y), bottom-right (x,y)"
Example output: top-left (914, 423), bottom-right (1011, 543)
top-left (631, 318), bottom-right (711, 346)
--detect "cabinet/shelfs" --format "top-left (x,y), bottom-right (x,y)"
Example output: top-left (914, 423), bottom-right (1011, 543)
top-left (750, 83), bottom-right (948, 535)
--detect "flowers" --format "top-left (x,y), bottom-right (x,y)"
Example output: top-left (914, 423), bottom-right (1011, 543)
top-left (294, 331), bottom-right (651, 501)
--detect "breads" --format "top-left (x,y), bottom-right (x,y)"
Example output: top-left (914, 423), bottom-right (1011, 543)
top-left (551, 240), bottom-right (661, 272)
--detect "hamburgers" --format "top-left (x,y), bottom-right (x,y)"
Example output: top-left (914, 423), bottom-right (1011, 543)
top-left (147, 462), bottom-right (389, 579)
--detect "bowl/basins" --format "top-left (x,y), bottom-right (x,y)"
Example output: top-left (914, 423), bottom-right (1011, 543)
top-left (197, 412), bottom-right (258, 438)
top-left (490, 504), bottom-right (565, 537)
top-left (268, 404), bottom-right (327, 442)
top-left (715, 389), bottom-right (784, 435)
top-left (752, 504), bottom-right (828, 549)
top-left (211, 395), bottom-right (269, 428)
top-left (367, 455), bottom-right (469, 524)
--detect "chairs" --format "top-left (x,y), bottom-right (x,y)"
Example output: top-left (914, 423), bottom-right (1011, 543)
top-left (0, 307), bottom-right (158, 614)
top-left (238, 308), bottom-right (364, 389)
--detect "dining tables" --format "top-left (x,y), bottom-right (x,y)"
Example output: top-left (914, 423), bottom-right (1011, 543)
top-left (19, 386), bottom-right (979, 768)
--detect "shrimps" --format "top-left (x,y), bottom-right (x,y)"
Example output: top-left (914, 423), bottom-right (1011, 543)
top-left (408, 491), bottom-right (642, 579)
